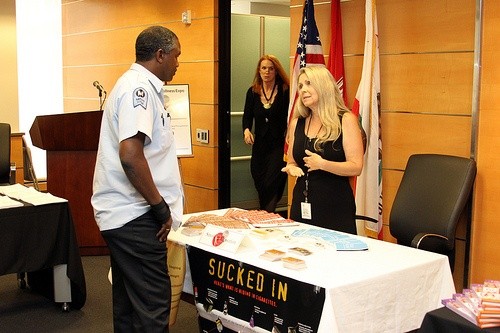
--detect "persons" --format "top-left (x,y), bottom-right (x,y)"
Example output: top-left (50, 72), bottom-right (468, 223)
top-left (243, 55), bottom-right (290, 213)
top-left (90, 25), bottom-right (184, 333)
top-left (280, 65), bottom-right (363, 235)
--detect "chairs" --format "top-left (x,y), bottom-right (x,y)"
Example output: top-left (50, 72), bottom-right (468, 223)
top-left (0, 123), bottom-right (10, 183)
top-left (388, 154), bottom-right (477, 273)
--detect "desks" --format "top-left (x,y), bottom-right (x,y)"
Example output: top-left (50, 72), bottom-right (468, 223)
top-left (0, 183), bottom-right (86, 314)
top-left (108, 207), bottom-right (456, 333)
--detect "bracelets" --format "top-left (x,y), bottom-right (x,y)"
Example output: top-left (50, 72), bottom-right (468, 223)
top-left (150, 196), bottom-right (170, 221)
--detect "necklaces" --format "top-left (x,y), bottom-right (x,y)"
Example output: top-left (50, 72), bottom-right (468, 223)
top-left (262, 83), bottom-right (277, 109)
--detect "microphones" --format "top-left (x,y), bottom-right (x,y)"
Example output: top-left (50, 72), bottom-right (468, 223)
top-left (93, 80), bottom-right (106, 93)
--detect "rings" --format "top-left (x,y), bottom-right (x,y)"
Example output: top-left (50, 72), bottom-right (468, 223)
top-left (166, 229), bottom-right (169, 230)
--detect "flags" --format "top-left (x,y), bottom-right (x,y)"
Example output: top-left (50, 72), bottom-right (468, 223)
top-left (327, 0), bottom-right (345, 108)
top-left (283, 0), bottom-right (325, 161)
top-left (353, 0), bottom-right (384, 240)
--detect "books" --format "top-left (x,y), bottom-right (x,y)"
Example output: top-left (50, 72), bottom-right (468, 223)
top-left (441, 280), bottom-right (500, 328)
